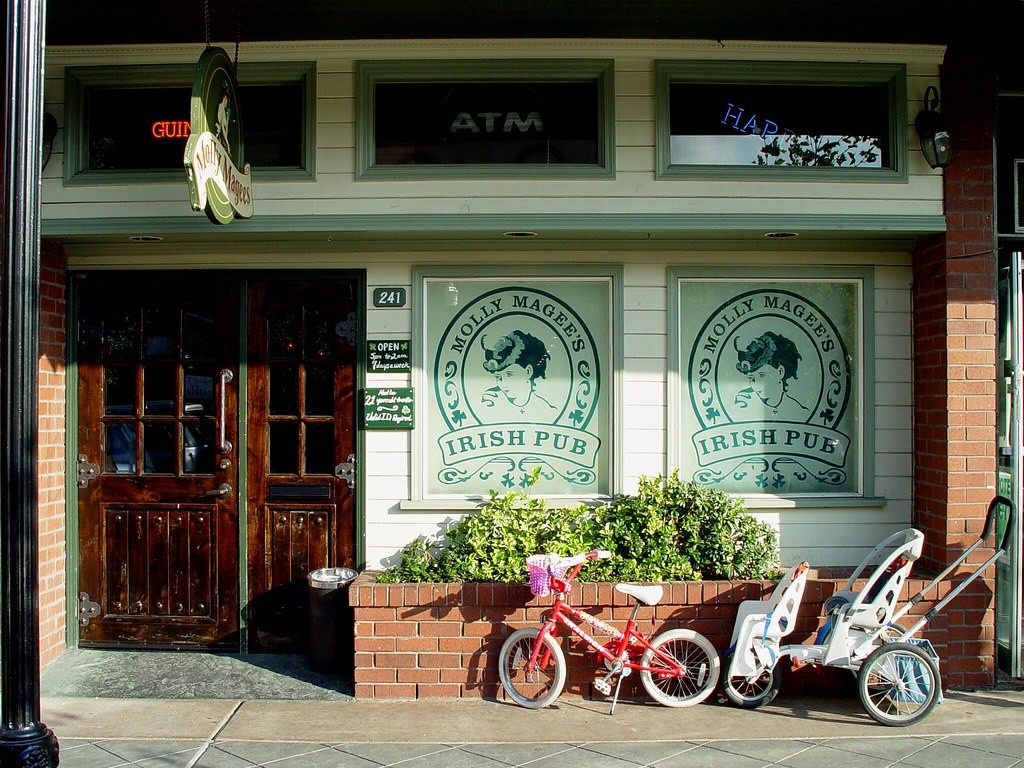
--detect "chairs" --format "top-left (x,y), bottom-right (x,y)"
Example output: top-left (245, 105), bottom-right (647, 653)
top-left (822, 528), bottom-right (925, 665)
top-left (729, 561), bottom-right (809, 677)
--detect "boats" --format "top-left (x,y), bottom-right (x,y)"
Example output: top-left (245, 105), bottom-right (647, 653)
top-left (496, 550), bottom-right (722, 716)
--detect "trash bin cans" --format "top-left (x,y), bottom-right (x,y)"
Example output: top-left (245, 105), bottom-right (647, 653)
top-left (306, 567), bottom-right (359, 676)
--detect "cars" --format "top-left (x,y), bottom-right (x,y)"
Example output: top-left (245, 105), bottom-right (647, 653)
top-left (106, 398), bottom-right (216, 475)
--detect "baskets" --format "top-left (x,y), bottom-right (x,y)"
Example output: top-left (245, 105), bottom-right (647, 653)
top-left (526, 554), bottom-right (570, 597)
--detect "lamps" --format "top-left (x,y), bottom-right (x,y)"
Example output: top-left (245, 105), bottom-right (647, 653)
top-left (915, 86), bottom-right (954, 169)
top-left (43, 112), bottom-right (57, 169)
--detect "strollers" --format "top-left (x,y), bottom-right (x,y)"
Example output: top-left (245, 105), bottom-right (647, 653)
top-left (719, 493), bottom-right (1016, 728)
